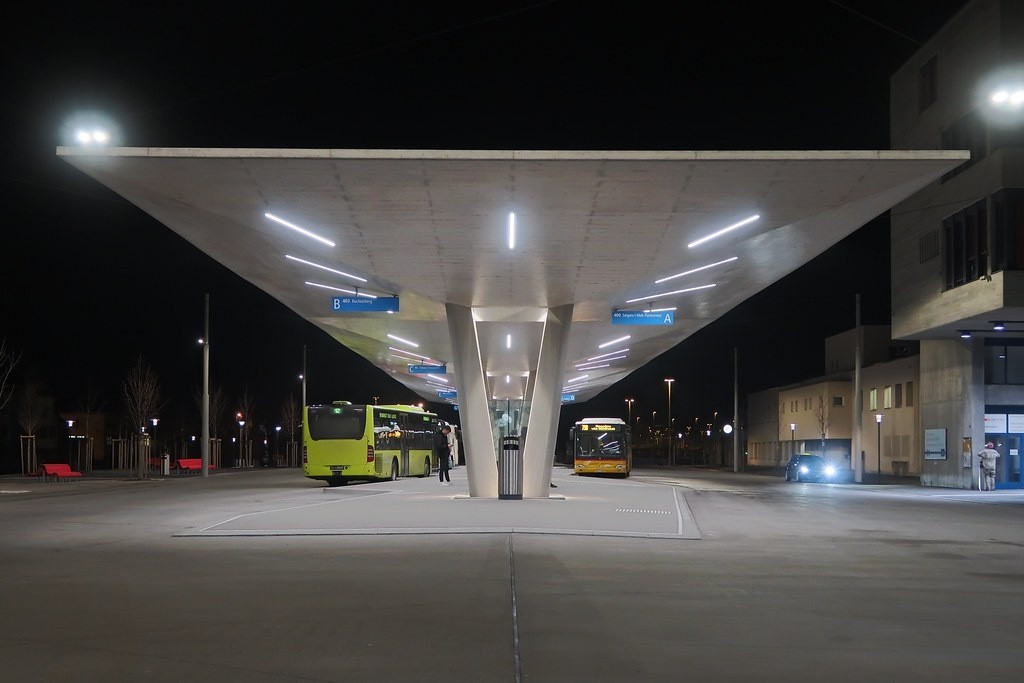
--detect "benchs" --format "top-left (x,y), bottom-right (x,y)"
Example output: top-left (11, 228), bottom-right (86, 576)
top-left (169, 459), bottom-right (216, 476)
top-left (148, 458), bottom-right (173, 472)
top-left (30, 464), bottom-right (82, 482)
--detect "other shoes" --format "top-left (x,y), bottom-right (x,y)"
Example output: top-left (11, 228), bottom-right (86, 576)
top-left (440, 482), bottom-right (453, 487)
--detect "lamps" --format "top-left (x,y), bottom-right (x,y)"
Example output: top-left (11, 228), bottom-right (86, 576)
top-left (960, 330), bottom-right (971, 338)
top-left (993, 321), bottom-right (1004, 330)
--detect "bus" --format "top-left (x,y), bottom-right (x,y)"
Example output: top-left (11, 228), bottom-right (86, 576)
top-left (432, 421), bottom-right (458, 472)
top-left (302, 401), bottom-right (445, 487)
top-left (569, 417), bottom-right (632, 478)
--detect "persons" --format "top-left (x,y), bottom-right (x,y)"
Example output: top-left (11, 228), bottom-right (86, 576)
top-left (976, 442), bottom-right (1000, 491)
top-left (432, 425), bottom-right (455, 485)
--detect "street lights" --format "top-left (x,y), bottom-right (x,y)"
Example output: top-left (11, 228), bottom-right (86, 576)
top-left (664, 377), bottom-right (675, 466)
top-left (789, 422), bottom-right (797, 457)
top-left (299, 344), bottom-right (307, 406)
top-left (874, 411), bottom-right (885, 480)
top-left (197, 293), bottom-right (211, 478)
top-left (624, 398), bottom-right (635, 425)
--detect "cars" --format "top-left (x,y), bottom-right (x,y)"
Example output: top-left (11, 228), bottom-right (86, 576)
top-left (786, 454), bottom-right (833, 484)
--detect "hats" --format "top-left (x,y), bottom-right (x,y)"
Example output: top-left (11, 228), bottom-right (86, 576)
top-left (988, 442), bottom-right (993, 448)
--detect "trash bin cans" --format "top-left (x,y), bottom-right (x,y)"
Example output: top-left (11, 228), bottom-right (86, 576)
top-left (161, 453), bottom-right (170, 478)
top-left (498, 435), bottom-right (523, 500)
